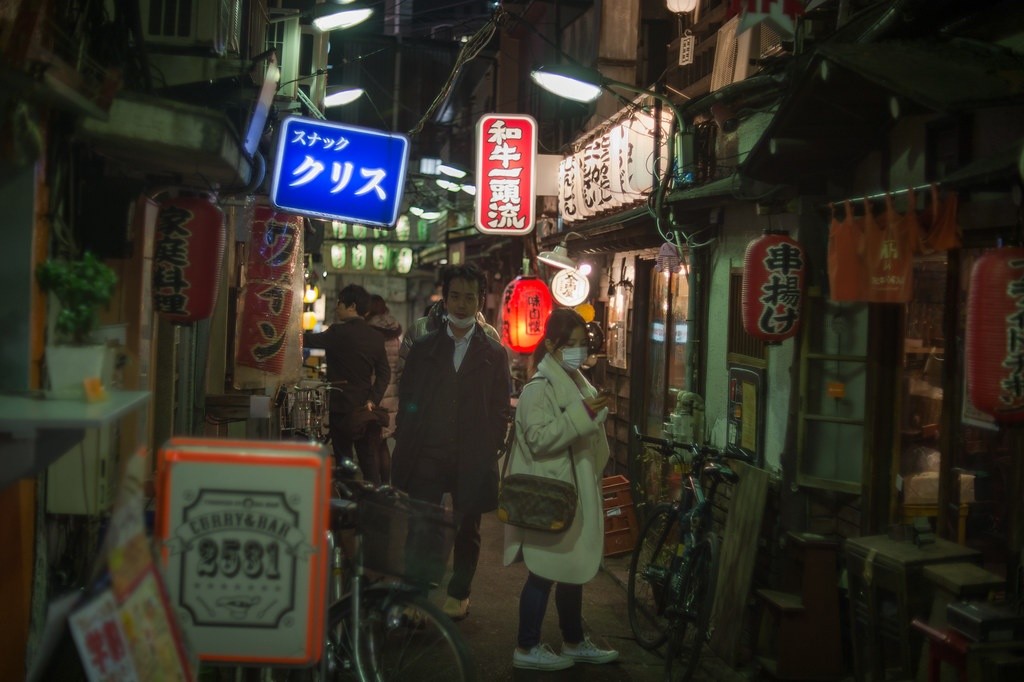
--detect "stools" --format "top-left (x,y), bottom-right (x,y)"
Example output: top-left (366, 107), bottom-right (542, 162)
top-left (902, 502), bottom-right (970, 546)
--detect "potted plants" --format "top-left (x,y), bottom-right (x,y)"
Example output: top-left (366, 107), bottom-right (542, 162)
top-left (34, 250), bottom-right (118, 401)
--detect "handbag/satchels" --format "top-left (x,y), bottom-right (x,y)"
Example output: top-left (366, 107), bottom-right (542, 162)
top-left (498, 474), bottom-right (576, 532)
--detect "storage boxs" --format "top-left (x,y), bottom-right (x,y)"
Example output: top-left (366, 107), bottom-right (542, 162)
top-left (602, 475), bottom-right (639, 558)
top-left (844, 533), bottom-right (972, 682)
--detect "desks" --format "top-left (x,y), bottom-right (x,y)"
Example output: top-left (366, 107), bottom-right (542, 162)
top-left (758, 529), bottom-right (847, 682)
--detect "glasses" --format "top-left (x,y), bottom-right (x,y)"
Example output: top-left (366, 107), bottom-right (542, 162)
top-left (338, 299), bottom-right (343, 305)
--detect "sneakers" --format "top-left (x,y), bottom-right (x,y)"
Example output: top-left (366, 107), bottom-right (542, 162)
top-left (513, 645), bottom-right (574, 671)
top-left (559, 636), bottom-right (618, 664)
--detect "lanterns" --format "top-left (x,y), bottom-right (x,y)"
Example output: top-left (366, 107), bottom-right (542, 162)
top-left (742, 229), bottom-right (805, 347)
top-left (966, 247), bottom-right (1024, 427)
top-left (134, 188), bottom-right (227, 326)
top-left (499, 274), bottom-right (552, 356)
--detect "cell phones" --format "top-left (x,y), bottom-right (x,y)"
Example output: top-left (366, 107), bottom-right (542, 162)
top-left (598, 388), bottom-right (612, 396)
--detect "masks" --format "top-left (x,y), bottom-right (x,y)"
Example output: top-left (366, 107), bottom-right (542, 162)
top-left (446, 314), bottom-right (476, 329)
top-left (561, 347), bottom-right (588, 373)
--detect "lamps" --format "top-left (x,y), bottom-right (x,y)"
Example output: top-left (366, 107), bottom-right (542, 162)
top-left (266, 0), bottom-right (376, 34)
top-left (322, 84), bottom-right (364, 108)
top-left (531, 64), bottom-right (695, 179)
top-left (536, 231), bottom-right (588, 272)
top-left (437, 162), bottom-right (474, 179)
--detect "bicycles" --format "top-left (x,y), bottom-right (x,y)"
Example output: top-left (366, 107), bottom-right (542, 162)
top-left (626, 422), bottom-right (765, 682)
top-left (262, 456), bottom-right (476, 682)
top-left (279, 363), bottom-right (348, 445)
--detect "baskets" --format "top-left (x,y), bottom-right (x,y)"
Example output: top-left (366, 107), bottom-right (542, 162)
top-left (383, 491), bottom-right (462, 586)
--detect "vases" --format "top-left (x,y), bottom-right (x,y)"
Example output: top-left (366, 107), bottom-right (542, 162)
top-left (87, 322), bottom-right (129, 368)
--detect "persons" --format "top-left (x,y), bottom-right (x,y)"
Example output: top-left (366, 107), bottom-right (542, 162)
top-left (303, 281), bottom-right (499, 531)
top-left (504, 307), bottom-right (620, 670)
top-left (391, 264), bottom-right (511, 627)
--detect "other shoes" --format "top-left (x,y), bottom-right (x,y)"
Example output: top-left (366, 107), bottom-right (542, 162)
top-left (441, 595), bottom-right (469, 619)
top-left (402, 607), bottom-right (426, 629)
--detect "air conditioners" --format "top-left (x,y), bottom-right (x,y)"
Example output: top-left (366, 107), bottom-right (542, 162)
top-left (264, 7), bottom-right (300, 103)
top-left (708, 12), bottom-right (760, 93)
top-left (759, 22), bottom-right (793, 59)
top-left (127, 0), bottom-right (229, 60)
